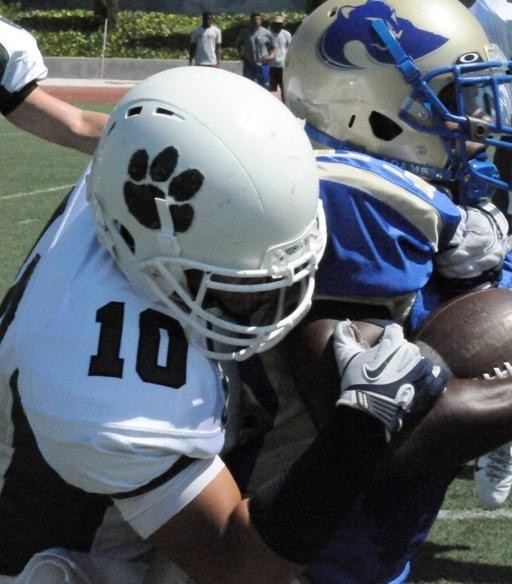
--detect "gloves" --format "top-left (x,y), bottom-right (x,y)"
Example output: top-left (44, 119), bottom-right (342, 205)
top-left (333, 318), bottom-right (449, 443)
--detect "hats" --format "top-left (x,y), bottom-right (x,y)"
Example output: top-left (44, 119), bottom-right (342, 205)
top-left (269, 16), bottom-right (286, 26)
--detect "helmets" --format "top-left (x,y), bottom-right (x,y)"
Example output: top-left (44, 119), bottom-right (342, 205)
top-left (89, 66), bottom-right (327, 362)
top-left (282, 0), bottom-right (512, 179)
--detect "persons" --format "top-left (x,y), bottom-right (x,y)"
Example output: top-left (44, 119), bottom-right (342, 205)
top-left (267, 13), bottom-right (293, 93)
top-left (0, 18), bottom-right (104, 152)
top-left (235, 12), bottom-right (277, 89)
top-left (0, 63), bottom-right (449, 582)
top-left (186, 10), bottom-right (223, 68)
top-left (277, 0), bottom-right (512, 479)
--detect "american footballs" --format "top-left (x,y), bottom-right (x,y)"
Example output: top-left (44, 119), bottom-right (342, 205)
top-left (412, 288), bottom-right (512, 380)
